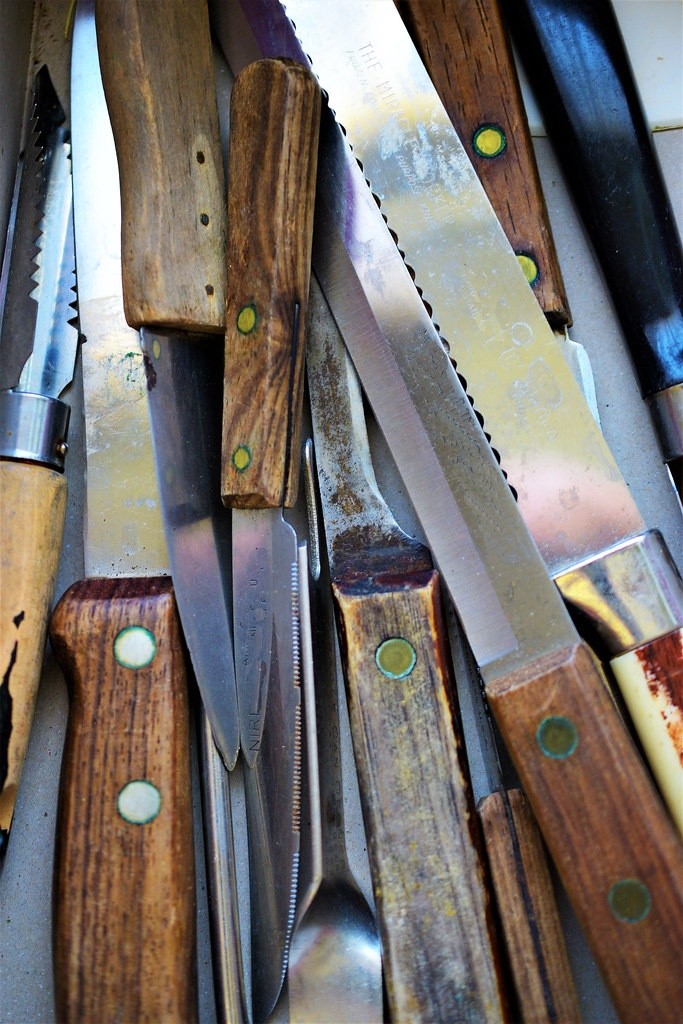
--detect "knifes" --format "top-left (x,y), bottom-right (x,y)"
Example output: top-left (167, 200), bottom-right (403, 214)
top-left (1, 0), bottom-right (683, 1023)
